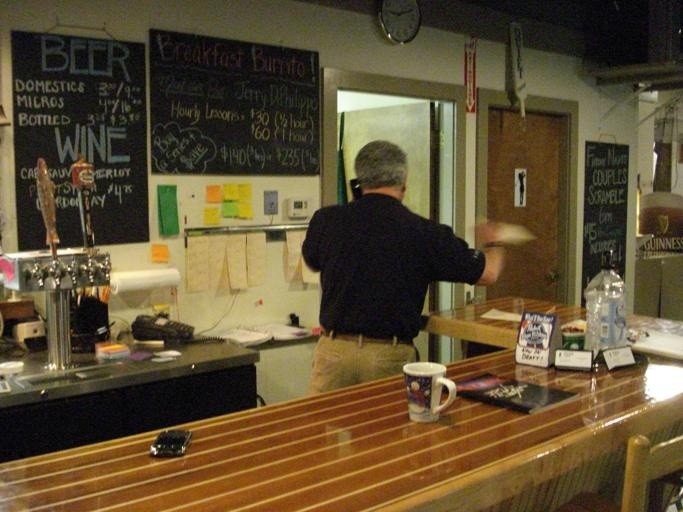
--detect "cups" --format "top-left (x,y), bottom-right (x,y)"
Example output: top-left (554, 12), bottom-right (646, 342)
top-left (402, 359), bottom-right (458, 423)
top-left (560, 323), bottom-right (585, 350)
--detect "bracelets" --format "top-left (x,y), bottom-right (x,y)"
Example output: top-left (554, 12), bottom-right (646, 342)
top-left (483, 240), bottom-right (507, 250)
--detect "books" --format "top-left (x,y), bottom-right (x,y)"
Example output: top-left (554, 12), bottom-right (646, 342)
top-left (629, 327), bottom-right (683, 363)
top-left (249, 321), bottom-right (314, 343)
top-left (440, 372), bottom-right (582, 416)
top-left (215, 329), bottom-right (274, 348)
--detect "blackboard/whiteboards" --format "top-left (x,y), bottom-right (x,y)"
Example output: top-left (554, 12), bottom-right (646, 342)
top-left (11, 30), bottom-right (149, 254)
top-left (150, 29), bottom-right (321, 173)
top-left (581, 141), bottom-right (629, 307)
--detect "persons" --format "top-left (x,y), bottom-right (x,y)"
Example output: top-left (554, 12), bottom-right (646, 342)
top-left (299, 139), bottom-right (512, 398)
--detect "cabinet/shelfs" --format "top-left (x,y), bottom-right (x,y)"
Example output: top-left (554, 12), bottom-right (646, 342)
top-left (634, 250), bottom-right (683, 322)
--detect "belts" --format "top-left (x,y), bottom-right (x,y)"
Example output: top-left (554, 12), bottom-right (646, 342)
top-left (322, 328), bottom-right (412, 345)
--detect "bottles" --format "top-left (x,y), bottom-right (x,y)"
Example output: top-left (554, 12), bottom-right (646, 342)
top-left (582, 248), bottom-right (629, 365)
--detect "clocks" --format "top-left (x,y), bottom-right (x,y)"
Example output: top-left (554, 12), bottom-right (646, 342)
top-left (376, 0), bottom-right (422, 46)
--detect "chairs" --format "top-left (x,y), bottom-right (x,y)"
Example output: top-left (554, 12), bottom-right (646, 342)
top-left (549, 435), bottom-right (683, 512)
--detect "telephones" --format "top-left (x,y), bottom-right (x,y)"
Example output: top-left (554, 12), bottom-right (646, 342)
top-left (132, 315), bottom-right (194, 342)
top-left (350, 179), bottom-right (362, 199)
